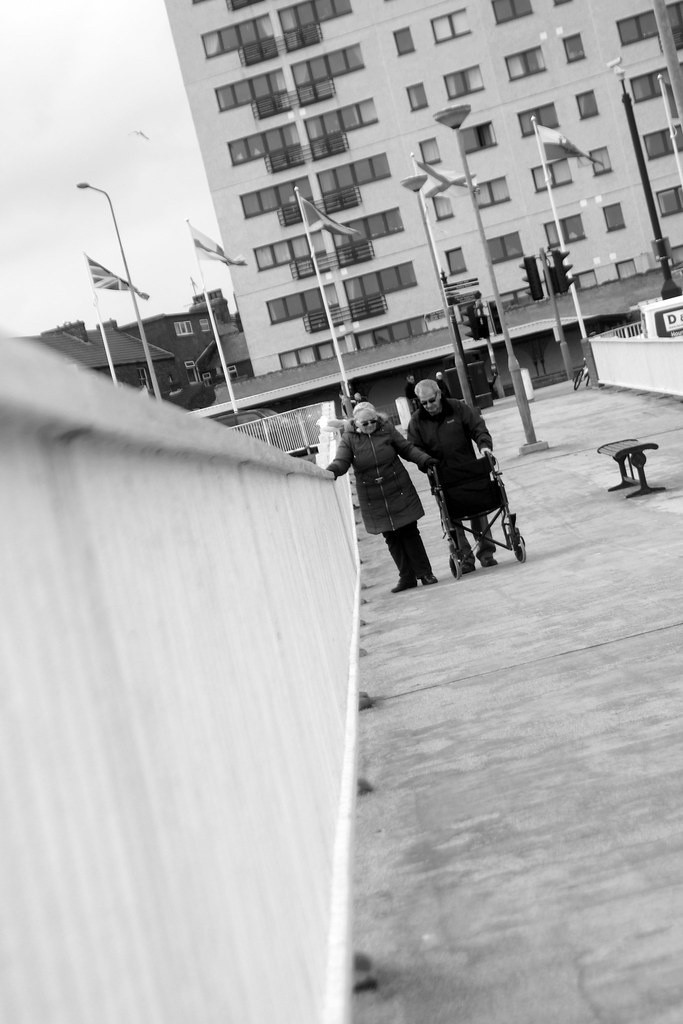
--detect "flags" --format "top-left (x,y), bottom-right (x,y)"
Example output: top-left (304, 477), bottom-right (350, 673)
top-left (190, 225), bottom-right (248, 267)
top-left (665, 83), bottom-right (679, 118)
top-left (536, 125), bottom-right (603, 166)
top-left (299, 195), bottom-right (361, 235)
top-left (86, 256), bottom-right (149, 299)
top-left (414, 158), bottom-right (478, 197)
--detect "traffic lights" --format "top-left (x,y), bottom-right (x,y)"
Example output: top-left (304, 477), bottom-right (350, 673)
top-left (551, 250), bottom-right (576, 293)
top-left (489, 300), bottom-right (503, 334)
top-left (461, 305), bottom-right (481, 340)
top-left (519, 257), bottom-right (544, 301)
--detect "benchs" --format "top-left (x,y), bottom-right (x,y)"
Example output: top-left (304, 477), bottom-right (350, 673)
top-left (597, 438), bottom-right (667, 499)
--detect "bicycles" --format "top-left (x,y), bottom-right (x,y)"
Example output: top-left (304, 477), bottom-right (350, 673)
top-left (574, 357), bottom-right (589, 390)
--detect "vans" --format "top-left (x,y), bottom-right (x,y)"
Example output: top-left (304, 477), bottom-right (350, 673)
top-left (212, 409), bottom-right (314, 457)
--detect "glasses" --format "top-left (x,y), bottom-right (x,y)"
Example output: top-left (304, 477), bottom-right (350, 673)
top-left (357, 417), bottom-right (377, 426)
top-left (420, 391), bottom-right (438, 405)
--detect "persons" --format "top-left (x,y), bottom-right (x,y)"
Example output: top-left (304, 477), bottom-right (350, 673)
top-left (405, 375), bottom-right (422, 413)
top-left (436, 372), bottom-right (451, 398)
top-left (327, 402), bottom-right (440, 593)
top-left (407, 380), bottom-right (498, 574)
top-left (339, 391), bottom-right (368, 415)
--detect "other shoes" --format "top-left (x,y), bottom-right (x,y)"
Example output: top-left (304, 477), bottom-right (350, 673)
top-left (481, 556), bottom-right (497, 567)
top-left (462, 562), bottom-right (475, 574)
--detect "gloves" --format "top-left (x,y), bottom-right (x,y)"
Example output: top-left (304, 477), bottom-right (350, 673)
top-left (424, 457), bottom-right (438, 472)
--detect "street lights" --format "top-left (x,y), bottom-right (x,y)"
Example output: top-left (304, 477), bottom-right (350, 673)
top-left (400, 174), bottom-right (475, 410)
top-left (433, 104), bottom-right (549, 455)
top-left (77, 183), bottom-right (162, 403)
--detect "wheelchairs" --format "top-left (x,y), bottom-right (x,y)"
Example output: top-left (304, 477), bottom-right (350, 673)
top-left (428, 451), bottom-right (527, 580)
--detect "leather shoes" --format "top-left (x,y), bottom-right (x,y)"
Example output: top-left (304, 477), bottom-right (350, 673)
top-left (421, 574), bottom-right (438, 585)
top-left (390, 578), bottom-right (417, 593)
